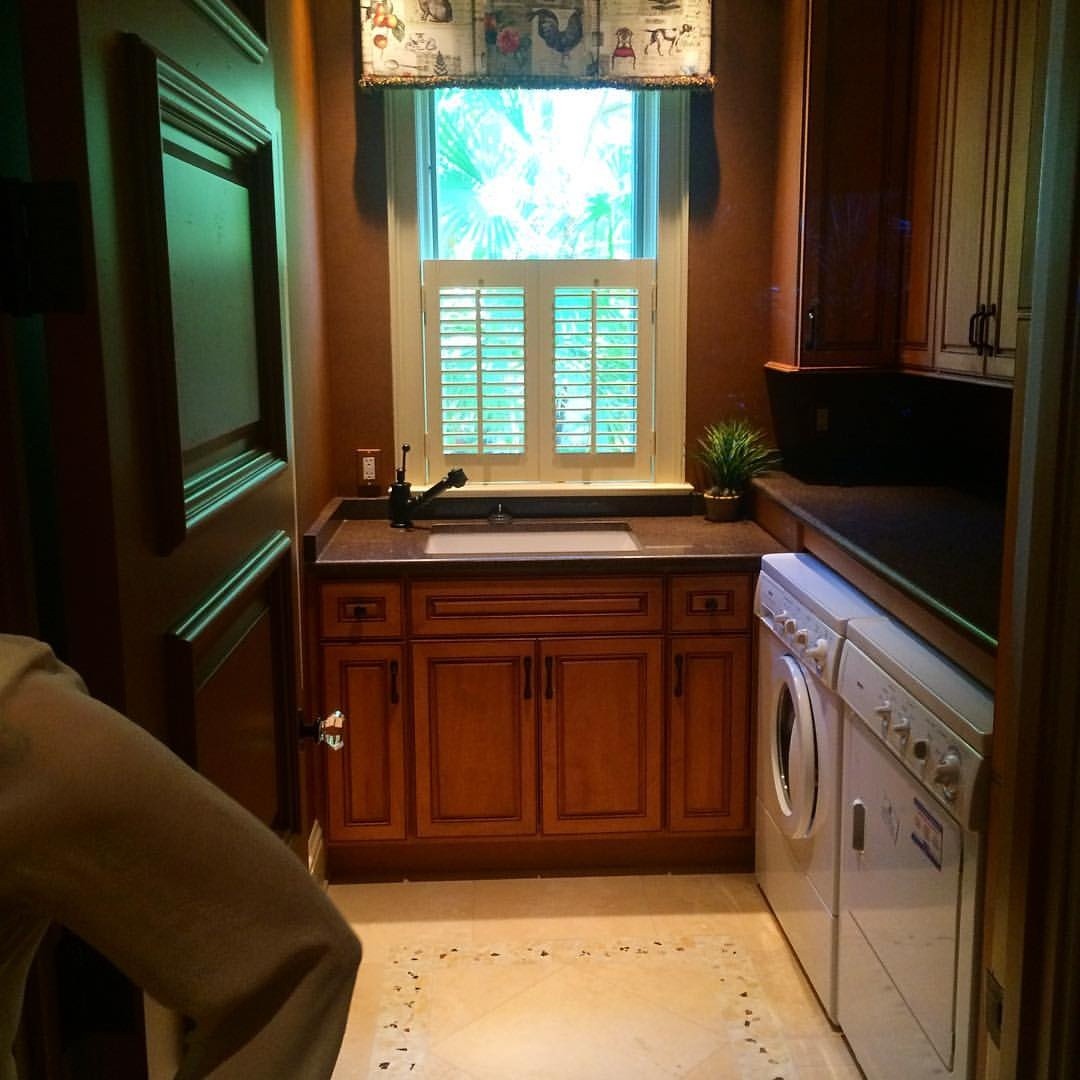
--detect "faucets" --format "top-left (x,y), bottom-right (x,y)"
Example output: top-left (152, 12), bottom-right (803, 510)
top-left (388, 443), bottom-right (469, 527)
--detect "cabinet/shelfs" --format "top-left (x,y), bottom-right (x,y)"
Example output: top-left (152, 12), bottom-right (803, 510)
top-left (664, 572), bottom-right (754, 876)
top-left (316, 575), bottom-right (405, 883)
top-left (765, 1), bottom-right (919, 377)
top-left (406, 575), bottom-right (667, 883)
top-left (897, 1), bottom-right (1037, 394)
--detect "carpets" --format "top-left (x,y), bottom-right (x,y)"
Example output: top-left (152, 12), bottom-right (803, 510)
top-left (364, 933), bottom-right (793, 1080)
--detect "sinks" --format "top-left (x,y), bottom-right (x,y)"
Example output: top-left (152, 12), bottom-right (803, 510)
top-left (422, 519), bottom-right (642, 554)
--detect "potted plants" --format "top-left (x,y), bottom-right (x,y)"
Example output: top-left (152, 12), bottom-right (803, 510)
top-left (691, 417), bottom-right (785, 523)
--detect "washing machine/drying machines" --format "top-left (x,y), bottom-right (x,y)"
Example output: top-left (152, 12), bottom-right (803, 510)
top-left (837, 616), bottom-right (995, 1080)
top-left (753, 550), bottom-right (889, 1028)
